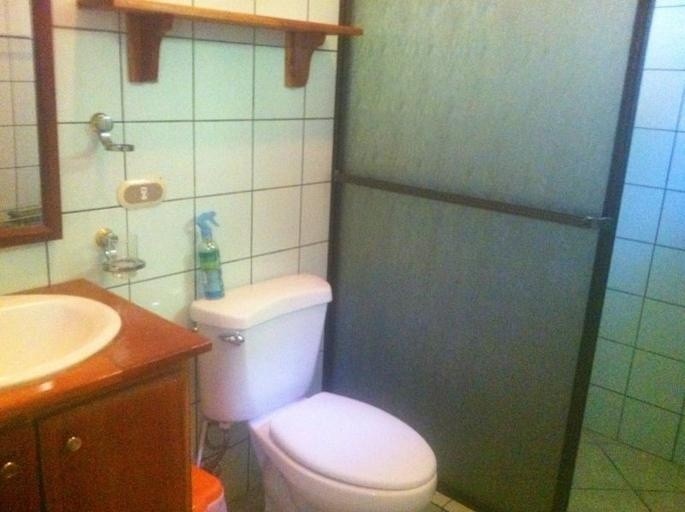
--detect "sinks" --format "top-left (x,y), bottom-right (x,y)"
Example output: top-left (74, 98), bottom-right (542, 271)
top-left (0, 294), bottom-right (123, 388)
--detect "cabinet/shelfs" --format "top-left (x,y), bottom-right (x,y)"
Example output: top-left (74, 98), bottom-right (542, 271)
top-left (0, 355), bottom-right (192, 512)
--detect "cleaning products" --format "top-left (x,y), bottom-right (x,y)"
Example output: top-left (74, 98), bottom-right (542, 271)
top-left (194, 211), bottom-right (224, 300)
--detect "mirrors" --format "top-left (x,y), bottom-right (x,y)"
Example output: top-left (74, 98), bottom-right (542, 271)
top-left (0, 0), bottom-right (63, 248)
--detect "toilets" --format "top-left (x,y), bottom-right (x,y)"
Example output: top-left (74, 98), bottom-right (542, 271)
top-left (177, 231), bottom-right (439, 512)
